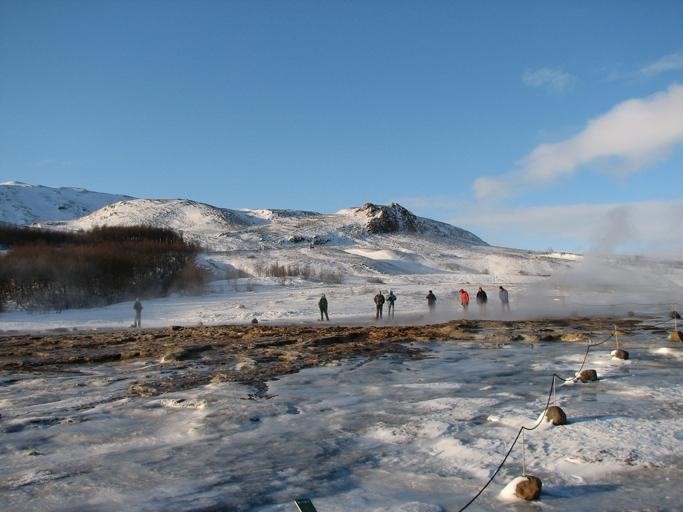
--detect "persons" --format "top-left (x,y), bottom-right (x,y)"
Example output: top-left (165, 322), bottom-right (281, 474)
top-left (425, 290), bottom-right (436, 313)
top-left (385, 290), bottom-right (396, 318)
top-left (497, 286), bottom-right (509, 311)
top-left (373, 290), bottom-right (384, 319)
top-left (458, 288), bottom-right (469, 310)
top-left (475, 287), bottom-right (487, 313)
top-left (317, 294), bottom-right (329, 321)
top-left (132, 297), bottom-right (142, 327)
